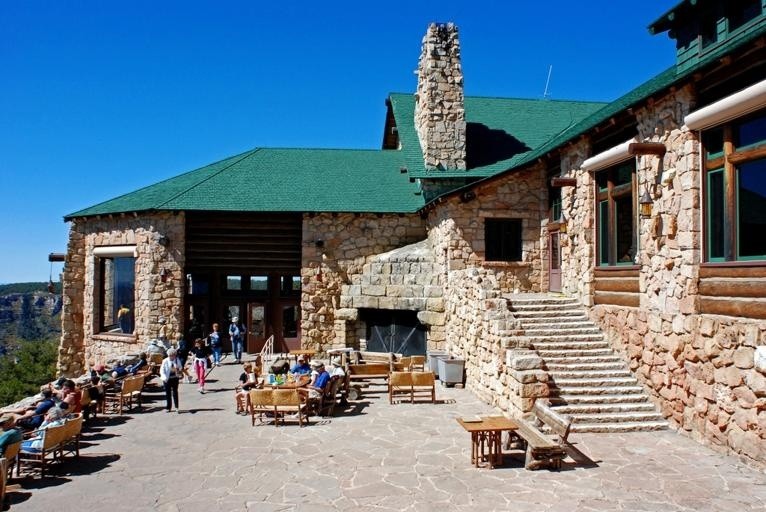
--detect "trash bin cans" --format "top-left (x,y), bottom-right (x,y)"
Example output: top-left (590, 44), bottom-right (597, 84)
top-left (428, 351), bottom-right (466, 388)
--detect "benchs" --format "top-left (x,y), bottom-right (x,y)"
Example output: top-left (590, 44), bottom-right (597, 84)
top-left (236, 347), bottom-right (435, 426)
top-left (504, 400), bottom-right (570, 472)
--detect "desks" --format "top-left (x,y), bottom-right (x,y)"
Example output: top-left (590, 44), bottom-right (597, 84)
top-left (455, 416), bottom-right (519, 471)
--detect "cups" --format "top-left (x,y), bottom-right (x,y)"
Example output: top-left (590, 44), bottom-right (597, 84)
top-left (267, 374), bottom-right (312, 386)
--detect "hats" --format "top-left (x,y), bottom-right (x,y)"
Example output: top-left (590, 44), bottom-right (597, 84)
top-left (297, 355), bottom-right (305, 362)
top-left (331, 357), bottom-right (342, 367)
top-left (232, 317), bottom-right (239, 324)
top-left (0, 414), bottom-right (14, 425)
top-left (95, 364), bottom-right (105, 372)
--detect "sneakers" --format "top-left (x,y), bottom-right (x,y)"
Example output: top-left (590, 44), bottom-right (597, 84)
top-left (292, 411), bottom-right (304, 419)
top-left (187, 375), bottom-right (206, 395)
top-left (212, 358), bottom-right (245, 367)
top-left (235, 408), bottom-right (250, 417)
top-left (165, 407), bottom-right (181, 414)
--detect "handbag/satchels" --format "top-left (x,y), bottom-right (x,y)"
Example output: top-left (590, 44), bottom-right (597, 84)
top-left (175, 357), bottom-right (185, 380)
top-left (207, 355), bottom-right (212, 369)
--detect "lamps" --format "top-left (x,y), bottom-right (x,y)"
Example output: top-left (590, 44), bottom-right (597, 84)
top-left (640, 156), bottom-right (653, 219)
top-left (160, 267), bottom-right (170, 282)
top-left (558, 208), bottom-right (568, 234)
top-left (316, 265), bottom-right (322, 281)
top-left (47, 261), bottom-right (54, 292)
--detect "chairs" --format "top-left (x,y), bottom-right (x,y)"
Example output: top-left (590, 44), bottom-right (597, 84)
top-left (0, 353), bottom-right (163, 511)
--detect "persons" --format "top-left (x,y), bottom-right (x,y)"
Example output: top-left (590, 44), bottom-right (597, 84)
top-left (235, 354), bottom-right (346, 420)
top-left (209, 322), bottom-right (222, 366)
top-left (0, 318), bottom-right (192, 459)
top-left (228, 317), bottom-right (246, 363)
top-left (118, 304), bottom-right (131, 334)
top-left (188, 337), bottom-right (213, 393)
